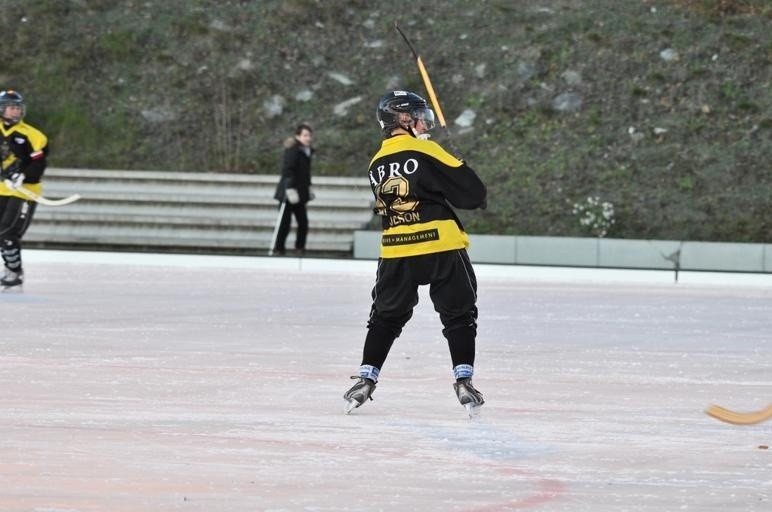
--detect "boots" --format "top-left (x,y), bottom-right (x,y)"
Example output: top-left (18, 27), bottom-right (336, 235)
top-left (0, 263), bottom-right (25, 285)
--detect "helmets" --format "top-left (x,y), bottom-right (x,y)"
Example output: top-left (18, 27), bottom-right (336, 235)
top-left (0, 90), bottom-right (26, 125)
top-left (375, 89), bottom-right (436, 140)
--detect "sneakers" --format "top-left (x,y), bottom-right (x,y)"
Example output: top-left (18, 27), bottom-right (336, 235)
top-left (272, 248), bottom-right (307, 257)
top-left (452, 377), bottom-right (486, 407)
top-left (344, 375), bottom-right (376, 408)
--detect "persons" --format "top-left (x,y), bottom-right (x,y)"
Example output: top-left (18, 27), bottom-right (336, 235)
top-left (0, 90), bottom-right (49, 286)
top-left (267, 125), bottom-right (316, 257)
top-left (343, 90), bottom-right (488, 408)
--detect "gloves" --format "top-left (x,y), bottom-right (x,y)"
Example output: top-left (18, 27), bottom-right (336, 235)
top-left (308, 186), bottom-right (315, 199)
top-left (285, 188), bottom-right (299, 203)
top-left (12, 172), bottom-right (25, 189)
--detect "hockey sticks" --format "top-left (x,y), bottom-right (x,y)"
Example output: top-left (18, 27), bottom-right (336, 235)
top-left (0, 170), bottom-right (82, 209)
top-left (394, 21), bottom-right (488, 216)
top-left (706, 402), bottom-right (772, 425)
